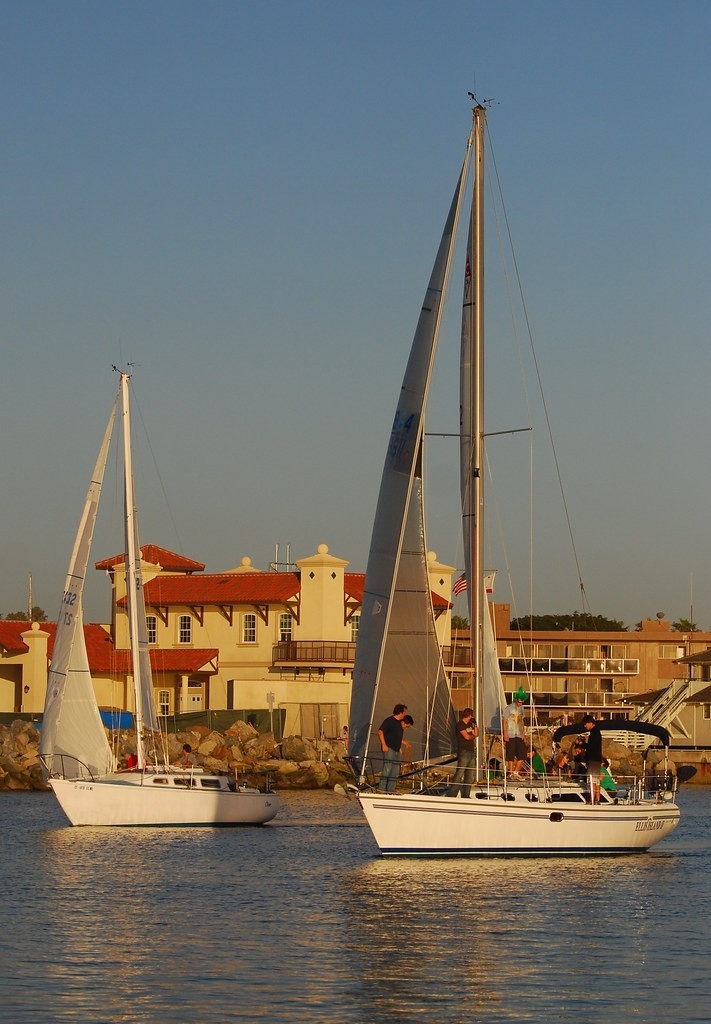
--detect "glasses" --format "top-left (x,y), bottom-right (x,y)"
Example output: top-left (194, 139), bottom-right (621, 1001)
top-left (517, 699), bottom-right (524, 704)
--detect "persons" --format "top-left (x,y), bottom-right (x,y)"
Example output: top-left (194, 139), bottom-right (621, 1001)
top-left (489, 758), bottom-right (502, 778)
top-left (339, 725), bottom-right (349, 738)
top-left (518, 745), bottom-right (544, 776)
top-left (572, 746), bottom-right (610, 778)
top-left (552, 751), bottom-right (572, 777)
top-left (124, 752), bottom-right (148, 768)
top-left (447, 708), bottom-right (479, 797)
top-left (573, 715), bottom-right (602, 804)
top-left (378, 704), bottom-right (407, 792)
top-left (503, 698), bottom-right (526, 780)
top-left (600, 756), bottom-right (617, 801)
top-left (174, 743), bottom-right (198, 769)
top-left (400, 715), bottom-right (414, 754)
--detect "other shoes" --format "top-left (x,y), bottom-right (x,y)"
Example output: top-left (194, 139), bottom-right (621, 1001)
top-left (508, 772), bottom-right (525, 781)
top-left (567, 779), bottom-right (580, 783)
top-left (587, 800), bottom-right (601, 805)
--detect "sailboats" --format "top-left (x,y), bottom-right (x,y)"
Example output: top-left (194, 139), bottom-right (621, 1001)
top-left (341, 87), bottom-right (682, 857)
top-left (35, 356), bottom-right (282, 828)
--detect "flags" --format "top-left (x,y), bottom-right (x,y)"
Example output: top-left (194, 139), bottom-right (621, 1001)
top-left (485, 572), bottom-right (495, 593)
top-left (452, 572), bottom-right (467, 596)
